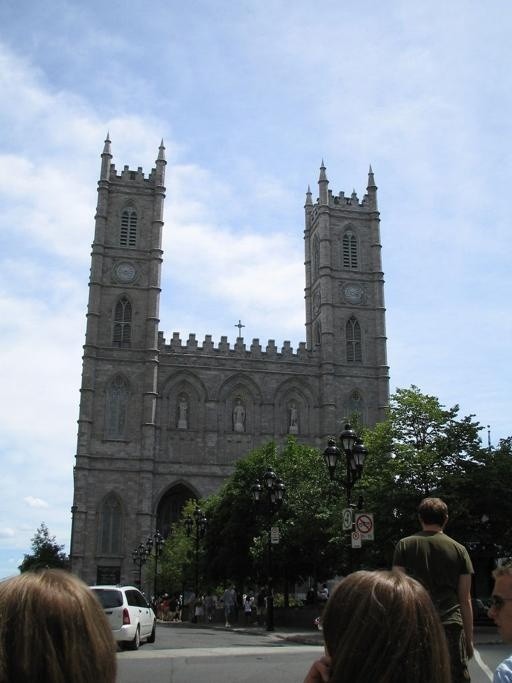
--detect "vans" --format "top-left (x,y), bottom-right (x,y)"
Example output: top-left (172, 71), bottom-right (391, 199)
top-left (84, 579), bottom-right (158, 653)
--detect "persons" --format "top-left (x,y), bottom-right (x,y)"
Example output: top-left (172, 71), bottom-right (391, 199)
top-left (319, 584), bottom-right (328, 599)
top-left (483, 554), bottom-right (512, 683)
top-left (177, 396), bottom-right (188, 428)
top-left (393, 498), bottom-right (474, 683)
top-left (233, 400), bottom-right (245, 432)
top-left (289, 403), bottom-right (298, 426)
top-left (303, 566), bottom-right (452, 683)
top-left (0, 566), bottom-right (117, 683)
top-left (151, 583), bottom-right (255, 628)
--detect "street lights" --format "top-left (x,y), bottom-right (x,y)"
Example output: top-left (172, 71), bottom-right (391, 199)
top-left (247, 467), bottom-right (288, 633)
top-left (146, 528), bottom-right (166, 606)
top-left (130, 542), bottom-right (149, 586)
top-left (183, 506), bottom-right (209, 628)
top-left (321, 420), bottom-right (370, 574)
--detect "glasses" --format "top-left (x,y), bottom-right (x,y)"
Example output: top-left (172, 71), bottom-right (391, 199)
top-left (486, 595), bottom-right (512, 609)
top-left (314, 616), bottom-right (325, 630)
